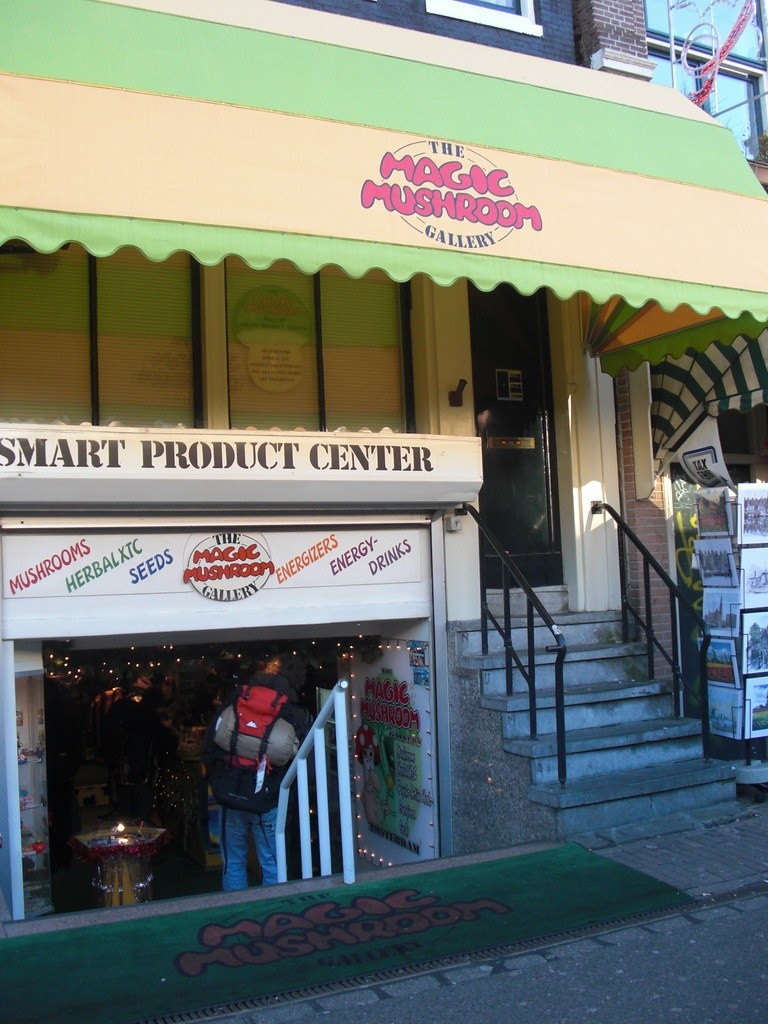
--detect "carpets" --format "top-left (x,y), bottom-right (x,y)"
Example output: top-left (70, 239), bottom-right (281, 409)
top-left (1, 840), bottom-right (700, 1022)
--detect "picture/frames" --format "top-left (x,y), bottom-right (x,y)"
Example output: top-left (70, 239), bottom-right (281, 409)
top-left (694, 483), bottom-right (768, 740)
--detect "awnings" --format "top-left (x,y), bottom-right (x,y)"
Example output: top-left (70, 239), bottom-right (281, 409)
top-left (0, 0), bottom-right (767, 499)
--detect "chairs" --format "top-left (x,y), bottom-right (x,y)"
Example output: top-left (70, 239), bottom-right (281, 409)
top-left (72, 763), bottom-right (109, 806)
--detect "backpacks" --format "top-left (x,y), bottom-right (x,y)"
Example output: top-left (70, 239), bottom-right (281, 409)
top-left (209, 675), bottom-right (304, 815)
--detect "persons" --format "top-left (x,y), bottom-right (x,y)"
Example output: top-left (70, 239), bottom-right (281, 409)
top-left (103, 639), bottom-right (338, 891)
top-left (698, 549), bottom-right (732, 579)
